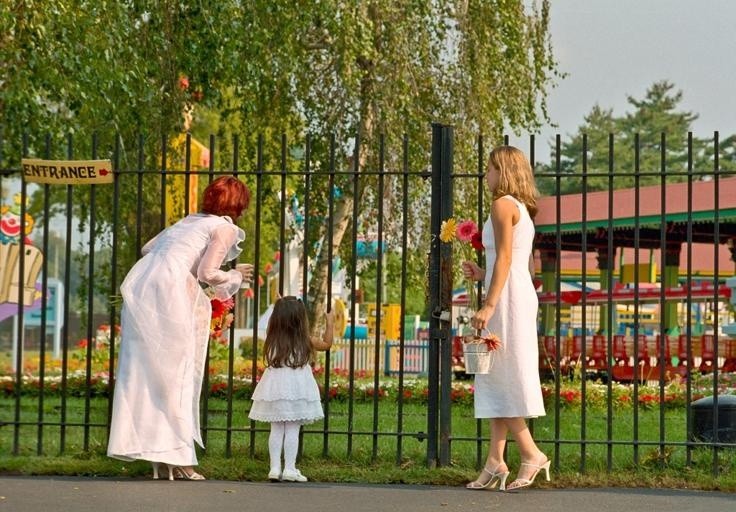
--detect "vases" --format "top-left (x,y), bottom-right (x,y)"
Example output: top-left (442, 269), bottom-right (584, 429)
top-left (462, 343), bottom-right (490, 375)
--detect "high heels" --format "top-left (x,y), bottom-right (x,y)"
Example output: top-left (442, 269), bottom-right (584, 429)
top-left (507, 460), bottom-right (552, 489)
top-left (466, 462), bottom-right (509, 490)
top-left (151, 461), bottom-right (206, 481)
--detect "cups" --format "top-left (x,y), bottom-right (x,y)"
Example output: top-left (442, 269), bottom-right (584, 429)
top-left (235, 264), bottom-right (250, 289)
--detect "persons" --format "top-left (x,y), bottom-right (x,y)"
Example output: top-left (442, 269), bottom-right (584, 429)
top-left (106, 177), bottom-right (254, 481)
top-left (465, 146), bottom-right (551, 491)
top-left (247, 295), bottom-right (337, 482)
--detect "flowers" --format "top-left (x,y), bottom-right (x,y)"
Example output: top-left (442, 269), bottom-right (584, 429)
top-left (439, 218), bottom-right (500, 350)
top-left (204, 286), bottom-right (235, 338)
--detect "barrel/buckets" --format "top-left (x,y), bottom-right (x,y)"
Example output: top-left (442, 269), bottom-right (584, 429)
top-left (461, 324), bottom-right (492, 374)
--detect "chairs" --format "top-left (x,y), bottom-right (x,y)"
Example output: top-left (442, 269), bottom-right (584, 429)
top-left (544, 334), bottom-right (736, 373)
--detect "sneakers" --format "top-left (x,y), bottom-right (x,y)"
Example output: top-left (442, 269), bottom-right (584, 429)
top-left (268, 468), bottom-right (282, 481)
top-left (283, 468), bottom-right (308, 482)
